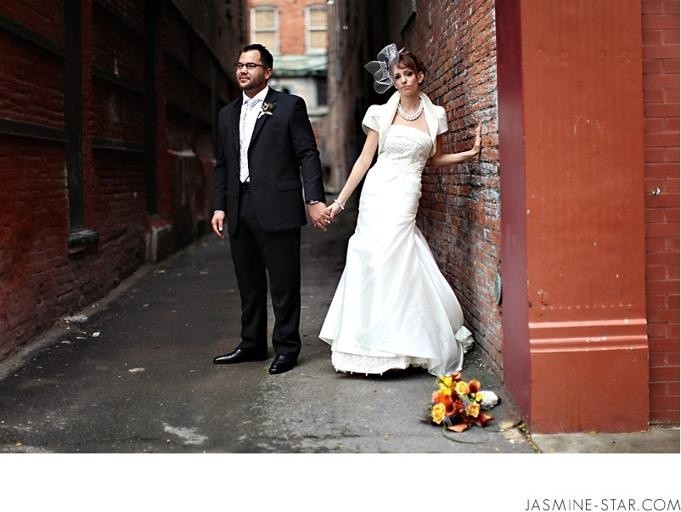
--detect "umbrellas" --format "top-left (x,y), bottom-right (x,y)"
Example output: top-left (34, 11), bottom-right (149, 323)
top-left (269, 354), bottom-right (299, 374)
top-left (213, 345), bottom-right (269, 364)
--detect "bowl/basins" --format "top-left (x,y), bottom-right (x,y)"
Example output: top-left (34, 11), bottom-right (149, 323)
top-left (395, 97), bottom-right (427, 123)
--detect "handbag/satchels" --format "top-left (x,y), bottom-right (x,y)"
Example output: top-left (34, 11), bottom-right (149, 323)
top-left (307, 200), bottom-right (320, 205)
top-left (333, 197), bottom-right (345, 212)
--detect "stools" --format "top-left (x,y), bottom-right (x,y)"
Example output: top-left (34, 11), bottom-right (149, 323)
top-left (240, 183), bottom-right (251, 192)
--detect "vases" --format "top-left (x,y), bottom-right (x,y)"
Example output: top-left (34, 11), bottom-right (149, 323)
top-left (419, 370), bottom-right (495, 434)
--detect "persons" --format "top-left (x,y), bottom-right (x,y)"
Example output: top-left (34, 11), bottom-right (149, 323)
top-left (208, 44), bottom-right (329, 374)
top-left (315, 44), bottom-right (483, 380)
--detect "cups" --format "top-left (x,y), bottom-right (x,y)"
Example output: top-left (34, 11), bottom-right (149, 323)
top-left (235, 62), bottom-right (265, 70)
